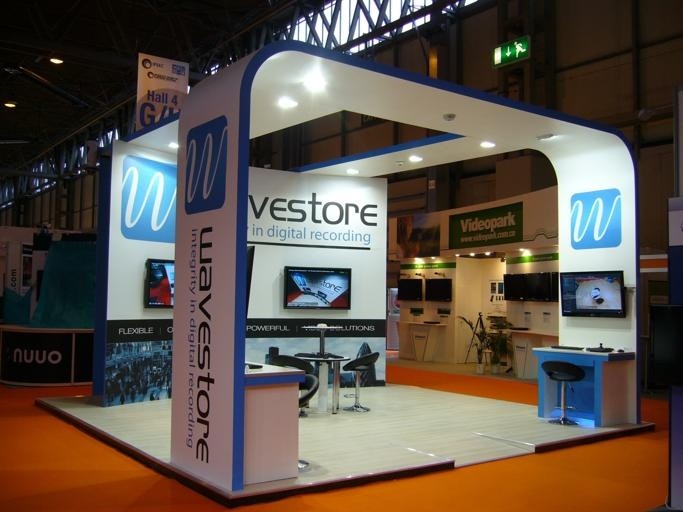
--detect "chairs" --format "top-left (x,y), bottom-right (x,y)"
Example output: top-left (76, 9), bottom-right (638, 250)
top-left (264, 346), bottom-right (313, 372)
top-left (297, 372), bottom-right (323, 471)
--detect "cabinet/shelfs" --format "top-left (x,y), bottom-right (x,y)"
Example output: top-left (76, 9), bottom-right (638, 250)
top-left (293, 325), bottom-right (346, 415)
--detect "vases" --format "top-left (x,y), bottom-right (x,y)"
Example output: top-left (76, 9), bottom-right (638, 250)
top-left (476, 364), bottom-right (484, 374)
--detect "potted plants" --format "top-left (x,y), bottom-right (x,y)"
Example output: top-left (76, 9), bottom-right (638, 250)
top-left (486, 331), bottom-right (512, 373)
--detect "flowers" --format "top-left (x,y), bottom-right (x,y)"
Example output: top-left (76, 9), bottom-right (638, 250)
top-left (459, 312), bottom-right (487, 364)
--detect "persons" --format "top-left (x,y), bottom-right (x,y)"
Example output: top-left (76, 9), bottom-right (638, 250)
top-left (104, 340), bottom-right (175, 404)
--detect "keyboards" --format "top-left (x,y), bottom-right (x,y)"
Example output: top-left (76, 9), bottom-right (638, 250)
top-left (551, 345), bottom-right (584, 350)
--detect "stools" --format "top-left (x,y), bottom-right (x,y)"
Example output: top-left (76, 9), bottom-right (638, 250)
top-left (539, 360), bottom-right (584, 427)
top-left (342, 351), bottom-right (380, 411)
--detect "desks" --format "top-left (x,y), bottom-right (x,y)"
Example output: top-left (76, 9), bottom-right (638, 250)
top-left (532, 345), bottom-right (636, 427)
top-left (243, 358), bottom-right (307, 485)
top-left (395, 321), bottom-right (448, 362)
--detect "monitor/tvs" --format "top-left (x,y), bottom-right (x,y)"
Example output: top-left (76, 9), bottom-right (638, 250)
top-left (398, 279), bottom-right (422, 301)
top-left (425, 278), bottom-right (452, 302)
top-left (560, 270), bottom-right (626, 316)
top-left (144, 258), bottom-right (175, 308)
top-left (283, 266), bottom-right (351, 309)
top-left (247, 246), bottom-right (255, 320)
top-left (504, 272), bottom-right (558, 302)
